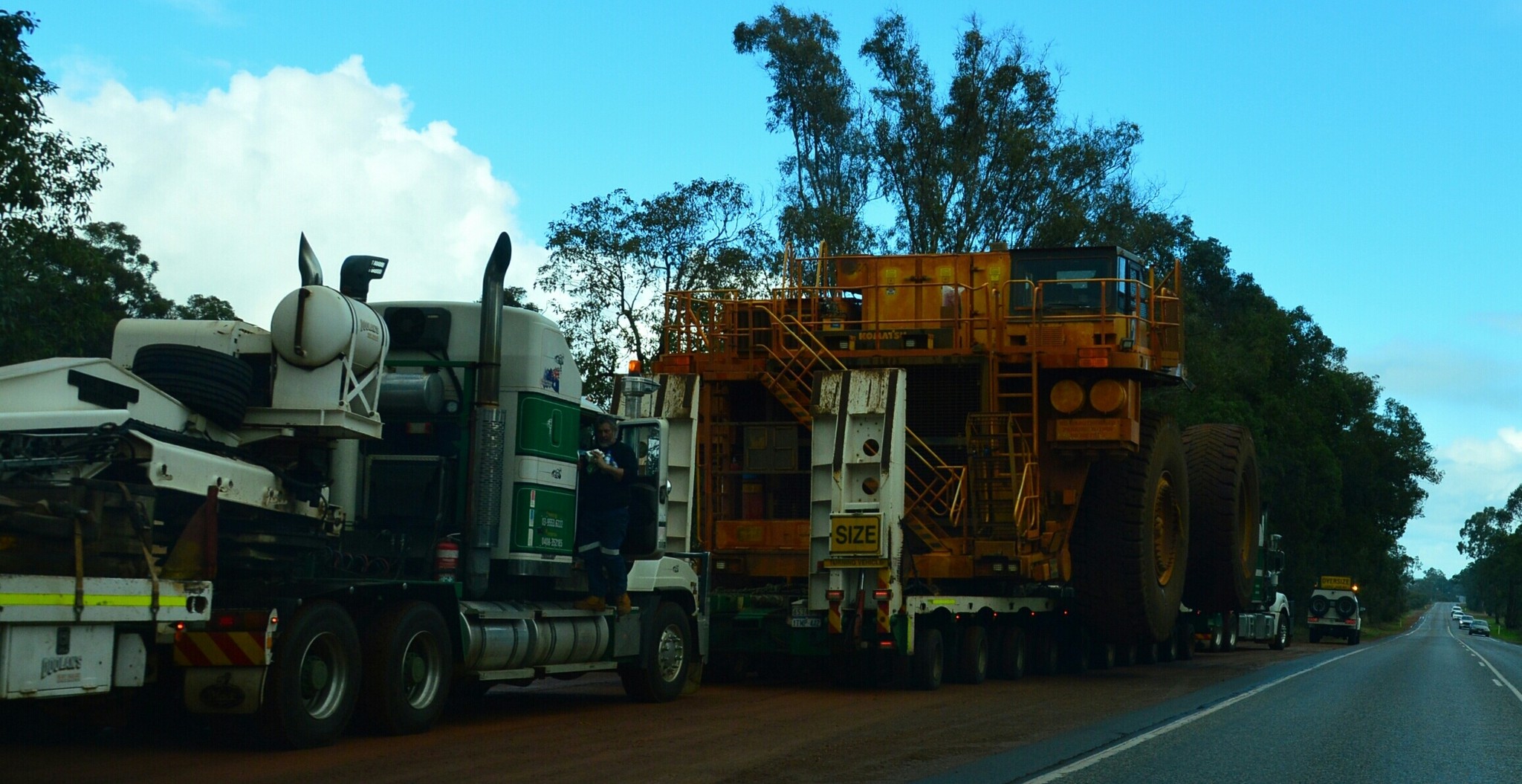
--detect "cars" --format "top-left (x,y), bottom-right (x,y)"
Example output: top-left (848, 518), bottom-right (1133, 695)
top-left (1458, 615), bottom-right (1474, 630)
top-left (1469, 619), bottom-right (1492, 637)
top-left (1452, 610), bottom-right (1464, 620)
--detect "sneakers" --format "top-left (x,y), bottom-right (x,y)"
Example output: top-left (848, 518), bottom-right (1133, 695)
top-left (575, 595), bottom-right (606, 612)
top-left (617, 593), bottom-right (631, 615)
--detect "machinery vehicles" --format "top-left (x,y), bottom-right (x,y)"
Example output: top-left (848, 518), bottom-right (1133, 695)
top-left (0, 229), bottom-right (667, 758)
top-left (652, 237), bottom-right (1263, 694)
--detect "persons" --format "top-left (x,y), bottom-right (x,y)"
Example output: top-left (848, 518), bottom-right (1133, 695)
top-left (577, 417), bottom-right (637, 616)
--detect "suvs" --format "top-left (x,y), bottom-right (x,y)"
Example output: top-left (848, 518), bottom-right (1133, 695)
top-left (1451, 606), bottom-right (1463, 617)
top-left (1305, 588), bottom-right (1367, 644)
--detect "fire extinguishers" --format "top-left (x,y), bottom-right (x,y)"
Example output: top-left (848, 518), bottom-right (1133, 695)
top-left (434, 532), bottom-right (461, 582)
